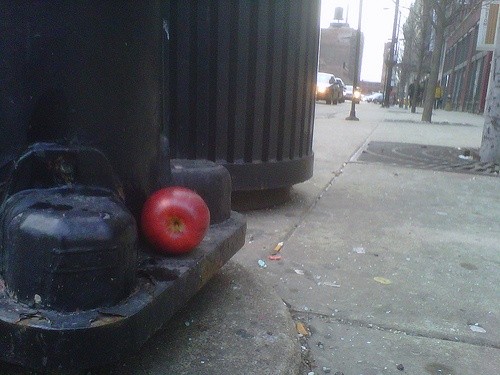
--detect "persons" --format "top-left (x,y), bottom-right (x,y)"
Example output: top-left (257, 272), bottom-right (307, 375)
top-left (433, 80), bottom-right (443, 110)
top-left (388, 79), bottom-right (424, 108)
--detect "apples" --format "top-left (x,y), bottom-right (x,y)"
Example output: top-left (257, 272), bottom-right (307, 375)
top-left (140, 185), bottom-right (210, 255)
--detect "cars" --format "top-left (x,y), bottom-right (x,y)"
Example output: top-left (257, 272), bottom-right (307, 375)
top-left (367, 92), bottom-right (383, 103)
top-left (317, 71), bottom-right (340, 103)
top-left (344, 85), bottom-right (353, 100)
top-left (335, 78), bottom-right (346, 102)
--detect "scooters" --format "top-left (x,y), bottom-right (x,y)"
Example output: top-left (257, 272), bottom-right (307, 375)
top-left (353, 92), bottom-right (361, 103)
